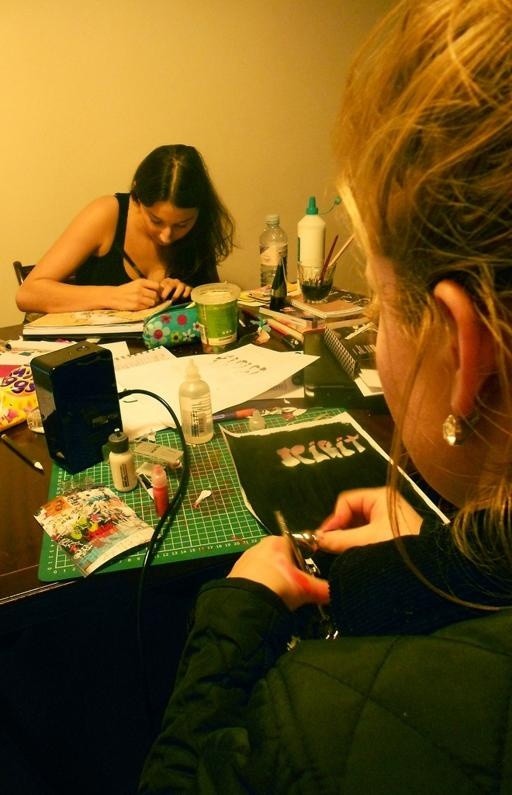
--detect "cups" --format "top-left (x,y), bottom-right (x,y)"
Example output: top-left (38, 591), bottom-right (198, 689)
top-left (295, 258), bottom-right (335, 303)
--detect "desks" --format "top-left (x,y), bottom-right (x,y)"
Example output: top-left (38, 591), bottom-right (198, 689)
top-left (0, 281), bottom-right (464, 628)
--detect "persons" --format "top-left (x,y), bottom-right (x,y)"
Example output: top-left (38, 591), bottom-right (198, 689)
top-left (138, 0), bottom-right (512, 795)
top-left (14, 144), bottom-right (234, 314)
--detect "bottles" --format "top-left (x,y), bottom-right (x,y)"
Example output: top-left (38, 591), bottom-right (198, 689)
top-left (258, 214), bottom-right (288, 286)
top-left (107, 432), bottom-right (137, 493)
top-left (179, 359), bottom-right (213, 444)
top-left (296, 195), bottom-right (342, 290)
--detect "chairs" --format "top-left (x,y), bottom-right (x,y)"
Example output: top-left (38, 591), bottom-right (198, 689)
top-left (12, 260), bottom-right (77, 320)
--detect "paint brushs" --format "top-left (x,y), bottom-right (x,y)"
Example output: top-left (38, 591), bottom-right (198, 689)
top-left (319, 234), bottom-right (339, 282)
top-left (328, 232), bottom-right (354, 268)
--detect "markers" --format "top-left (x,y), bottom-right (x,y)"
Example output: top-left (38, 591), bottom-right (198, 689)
top-left (211, 408), bottom-right (255, 421)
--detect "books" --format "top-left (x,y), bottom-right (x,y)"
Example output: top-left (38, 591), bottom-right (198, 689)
top-left (22, 299), bottom-right (175, 339)
top-left (237, 282), bottom-right (371, 343)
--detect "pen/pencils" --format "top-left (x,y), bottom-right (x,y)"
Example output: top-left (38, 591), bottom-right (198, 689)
top-left (117, 246), bottom-right (145, 279)
top-left (1, 432), bottom-right (44, 471)
